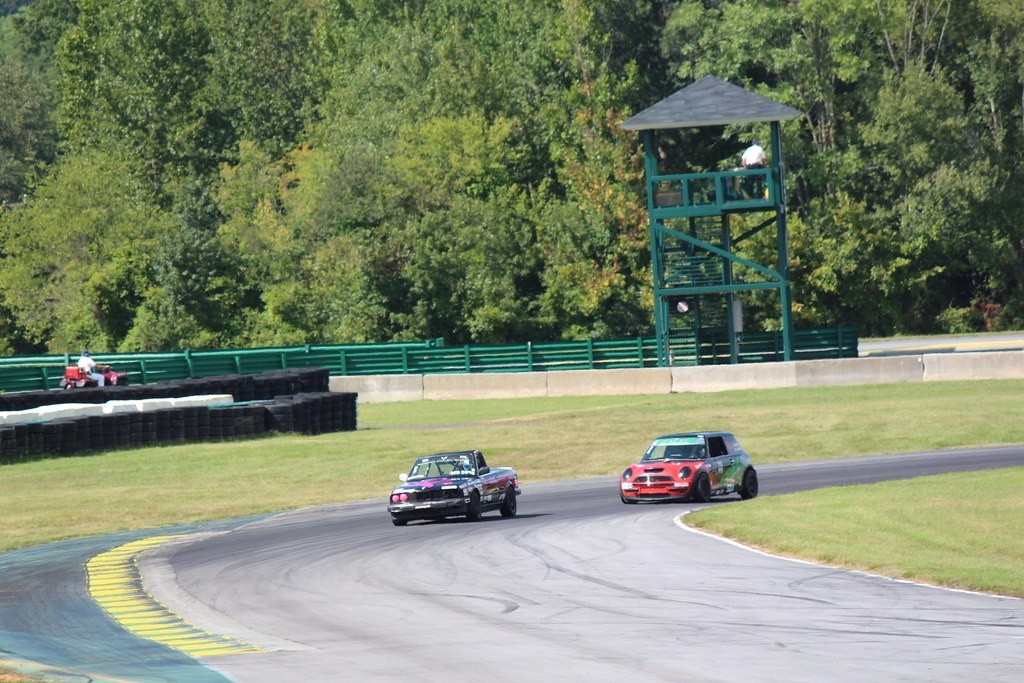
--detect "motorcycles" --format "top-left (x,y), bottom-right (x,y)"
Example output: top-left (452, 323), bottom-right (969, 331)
top-left (60, 364), bottom-right (128, 390)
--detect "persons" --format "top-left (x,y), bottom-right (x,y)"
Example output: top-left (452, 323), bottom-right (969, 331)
top-left (78, 350), bottom-right (105, 386)
top-left (688, 445), bottom-right (706, 458)
top-left (449, 455), bottom-right (475, 475)
top-left (742, 136), bottom-right (765, 199)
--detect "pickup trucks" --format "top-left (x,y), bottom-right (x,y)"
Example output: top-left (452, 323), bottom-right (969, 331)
top-left (387, 450), bottom-right (522, 526)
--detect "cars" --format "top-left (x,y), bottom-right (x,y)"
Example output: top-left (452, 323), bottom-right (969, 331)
top-left (619, 430), bottom-right (758, 505)
